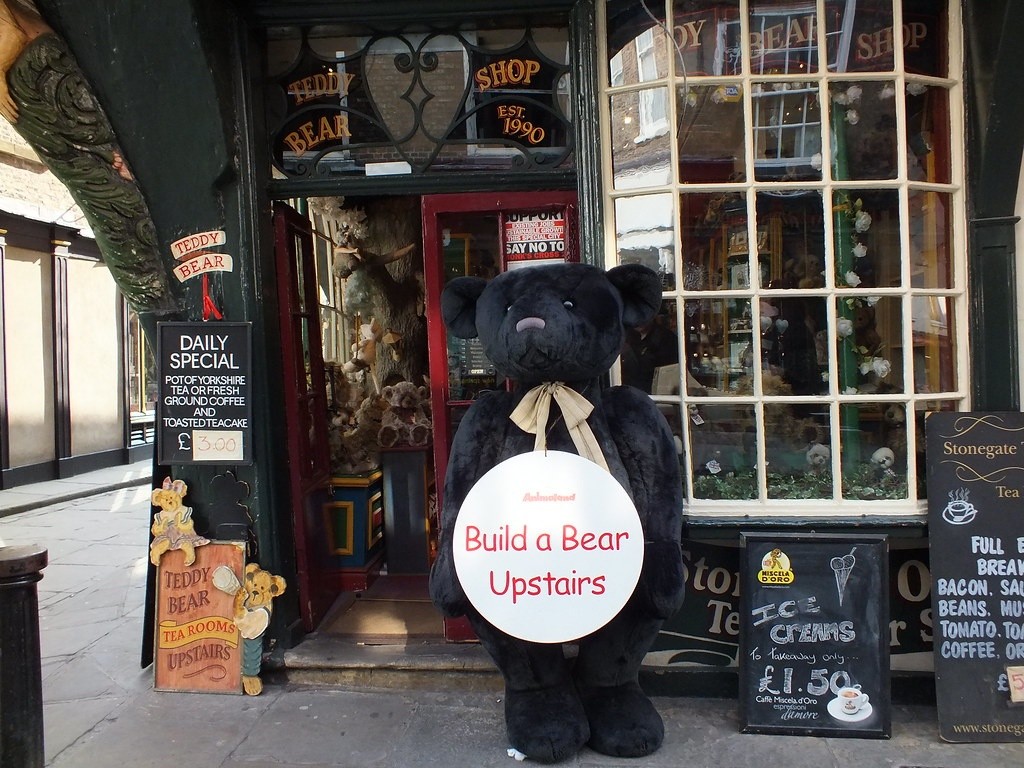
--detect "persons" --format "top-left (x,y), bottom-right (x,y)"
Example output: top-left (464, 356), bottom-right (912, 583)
top-left (628, 324), bottom-right (678, 395)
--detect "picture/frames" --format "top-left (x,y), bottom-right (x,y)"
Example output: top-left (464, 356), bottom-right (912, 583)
top-left (739, 531), bottom-right (891, 741)
top-left (157, 322), bottom-right (254, 466)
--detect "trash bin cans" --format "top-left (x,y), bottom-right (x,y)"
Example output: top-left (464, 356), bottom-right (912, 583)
top-left (0, 544), bottom-right (50, 766)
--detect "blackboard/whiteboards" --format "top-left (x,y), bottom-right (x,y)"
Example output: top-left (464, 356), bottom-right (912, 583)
top-left (924, 409), bottom-right (1024, 744)
top-left (155, 321), bottom-right (253, 466)
top-left (738, 530), bottom-right (893, 739)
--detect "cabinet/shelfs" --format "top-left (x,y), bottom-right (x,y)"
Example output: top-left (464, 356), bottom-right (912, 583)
top-left (693, 214), bottom-right (824, 392)
top-left (875, 88), bottom-right (954, 412)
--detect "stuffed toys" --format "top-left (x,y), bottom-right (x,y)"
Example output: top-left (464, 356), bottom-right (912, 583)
top-left (871, 446), bottom-right (895, 481)
top-left (670, 387), bottom-right (728, 474)
top-left (854, 306), bottom-right (881, 356)
top-left (378, 381), bottom-right (431, 449)
top-left (342, 318), bottom-right (375, 373)
top-left (207, 470), bottom-right (258, 555)
top-left (429, 263), bottom-right (685, 765)
top-left (816, 327), bottom-right (827, 365)
top-left (343, 397), bottom-right (388, 441)
top-left (807, 441), bottom-right (831, 479)
top-left (233, 563), bottom-right (287, 696)
top-left (150, 477), bottom-right (210, 566)
